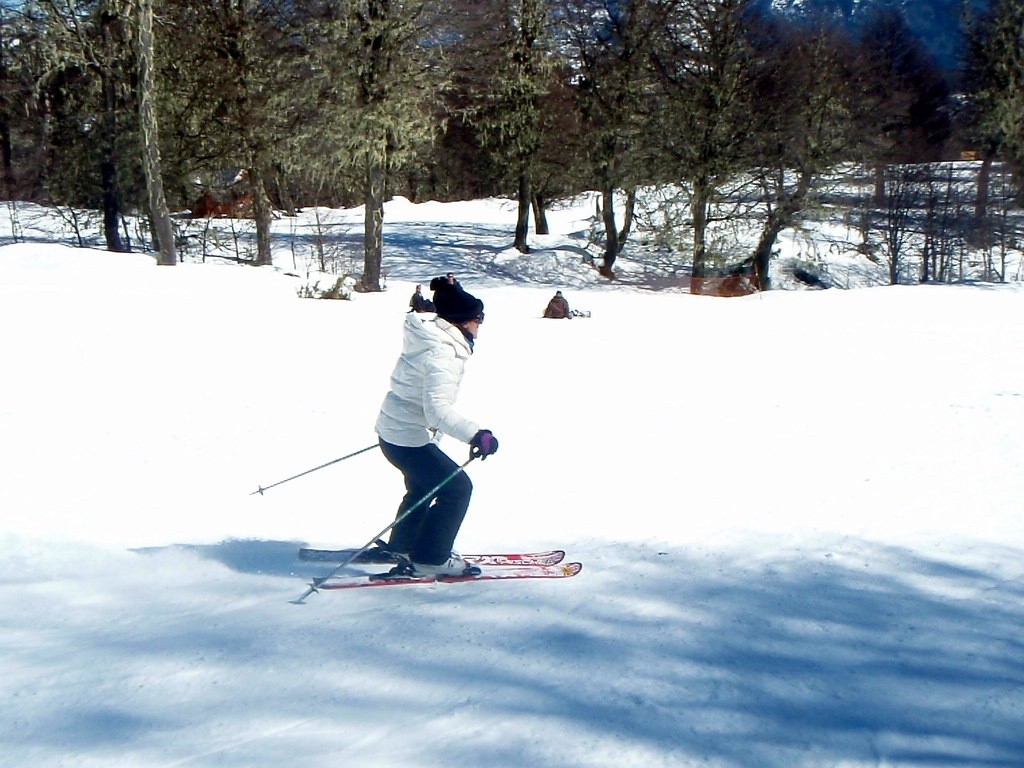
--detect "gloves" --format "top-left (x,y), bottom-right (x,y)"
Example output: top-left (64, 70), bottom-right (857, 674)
top-left (469, 429), bottom-right (499, 461)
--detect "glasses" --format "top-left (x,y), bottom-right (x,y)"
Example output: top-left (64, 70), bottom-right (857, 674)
top-left (476, 311), bottom-right (485, 324)
top-left (448, 276), bottom-right (454, 279)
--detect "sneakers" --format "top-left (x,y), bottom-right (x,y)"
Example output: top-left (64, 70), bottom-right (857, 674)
top-left (385, 539), bottom-right (481, 577)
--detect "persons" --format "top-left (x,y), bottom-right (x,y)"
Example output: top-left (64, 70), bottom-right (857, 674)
top-left (445, 271), bottom-right (464, 291)
top-left (409, 284), bottom-right (434, 313)
top-left (375, 276), bottom-right (498, 575)
top-left (541, 290), bottom-right (574, 320)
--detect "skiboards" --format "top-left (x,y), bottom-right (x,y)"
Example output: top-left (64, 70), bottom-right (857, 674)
top-left (299, 547), bottom-right (584, 589)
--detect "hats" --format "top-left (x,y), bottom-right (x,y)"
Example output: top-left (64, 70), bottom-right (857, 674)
top-left (432, 283), bottom-right (484, 325)
